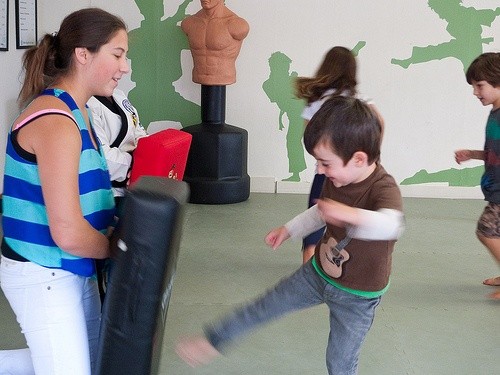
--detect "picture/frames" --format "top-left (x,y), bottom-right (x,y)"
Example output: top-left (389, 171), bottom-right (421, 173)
top-left (15, 0), bottom-right (37, 49)
top-left (0, 0), bottom-right (9, 51)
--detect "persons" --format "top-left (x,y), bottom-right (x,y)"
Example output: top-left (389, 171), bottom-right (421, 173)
top-left (454, 52), bottom-right (500, 301)
top-left (0, 7), bottom-right (130, 375)
top-left (180, 0), bottom-right (250, 86)
top-left (173, 95), bottom-right (405, 375)
top-left (292, 47), bottom-right (374, 265)
top-left (86, 89), bottom-right (149, 223)
